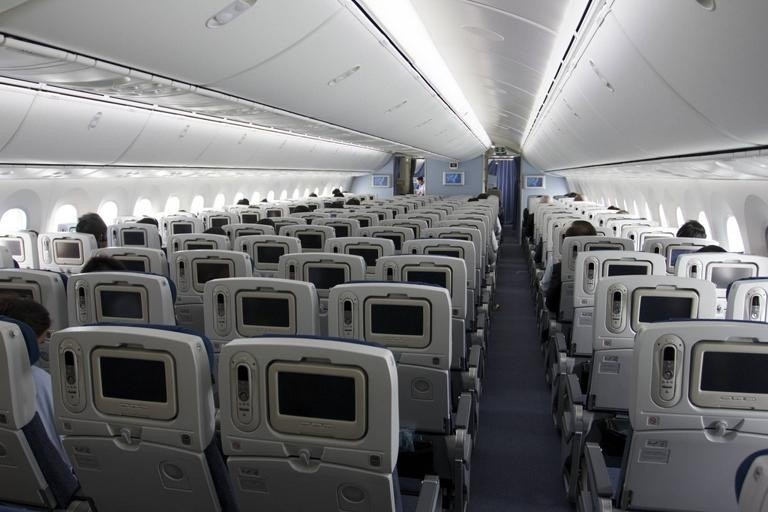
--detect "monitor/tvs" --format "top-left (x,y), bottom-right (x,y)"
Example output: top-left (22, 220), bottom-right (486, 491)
top-left (268, 362), bottom-right (366, 440)
top-left (559, 231), bottom-right (768, 410)
top-left (443, 171), bottom-right (465, 186)
top-left (524, 176), bottom-right (546, 189)
top-left (91, 348), bottom-right (178, 421)
top-left (371, 175), bottom-right (391, 188)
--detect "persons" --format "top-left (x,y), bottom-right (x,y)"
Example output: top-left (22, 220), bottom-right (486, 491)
top-left (146, 272), bottom-right (179, 324)
top-left (308, 188), bottom-right (348, 199)
top-left (204, 226), bottom-right (227, 237)
top-left (78, 256), bottom-right (128, 297)
top-left (258, 197), bottom-right (268, 204)
top-left (136, 216), bottom-right (170, 249)
top-left (0, 289), bottom-right (79, 511)
top-left (236, 198), bottom-right (249, 206)
top-left (258, 216), bottom-right (275, 227)
top-left (521, 191), bottom-right (741, 319)
top-left (470, 186), bottom-right (502, 252)
top-left (415, 177), bottom-right (424, 196)
top-left (74, 214), bottom-right (108, 251)
